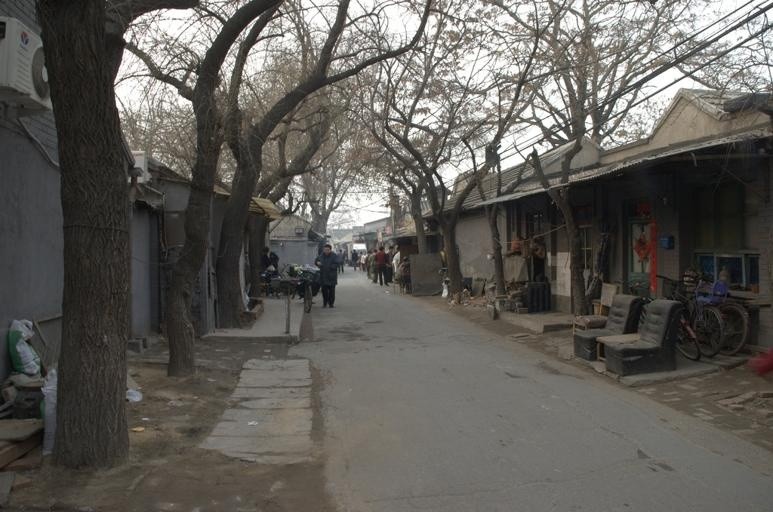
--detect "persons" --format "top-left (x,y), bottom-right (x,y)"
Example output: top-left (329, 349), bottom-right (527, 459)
top-left (262, 241), bottom-right (411, 309)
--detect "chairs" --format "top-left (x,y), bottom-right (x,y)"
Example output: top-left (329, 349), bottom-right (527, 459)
top-left (572, 294), bottom-right (683, 375)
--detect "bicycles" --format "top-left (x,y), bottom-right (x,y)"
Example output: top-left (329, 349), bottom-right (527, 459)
top-left (296, 267), bottom-right (317, 313)
top-left (653, 274), bottom-right (751, 361)
